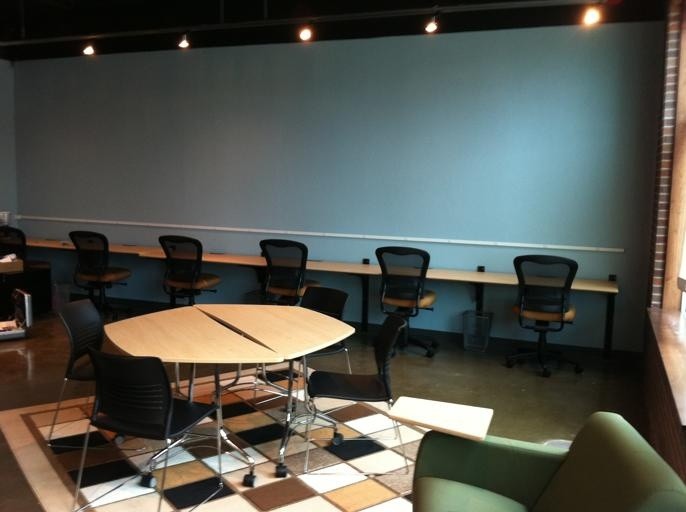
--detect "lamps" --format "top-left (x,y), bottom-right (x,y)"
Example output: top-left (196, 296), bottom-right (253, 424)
top-left (423, 14), bottom-right (440, 34)
top-left (176, 32), bottom-right (192, 49)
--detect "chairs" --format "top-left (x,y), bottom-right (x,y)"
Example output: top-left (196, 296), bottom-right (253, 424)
top-left (69, 345), bottom-right (225, 511)
top-left (301, 312), bottom-right (409, 477)
top-left (156, 232), bottom-right (220, 305)
top-left (497, 250), bottom-right (587, 379)
top-left (259, 237), bottom-right (307, 305)
top-left (45, 298), bottom-right (148, 453)
top-left (64, 228), bottom-right (132, 321)
top-left (410, 406), bottom-right (686, 511)
top-left (372, 244), bottom-right (442, 361)
top-left (252, 285), bottom-right (354, 410)
top-left (0, 223), bottom-right (53, 308)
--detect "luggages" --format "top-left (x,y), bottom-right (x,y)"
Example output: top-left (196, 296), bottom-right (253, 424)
top-left (0, 289), bottom-right (33, 340)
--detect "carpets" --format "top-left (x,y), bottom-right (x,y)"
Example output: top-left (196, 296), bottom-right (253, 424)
top-left (0, 357), bottom-right (479, 511)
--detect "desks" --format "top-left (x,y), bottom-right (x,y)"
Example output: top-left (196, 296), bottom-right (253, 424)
top-left (23, 239), bottom-right (624, 372)
top-left (195, 298), bottom-right (359, 480)
top-left (101, 302), bottom-right (283, 501)
